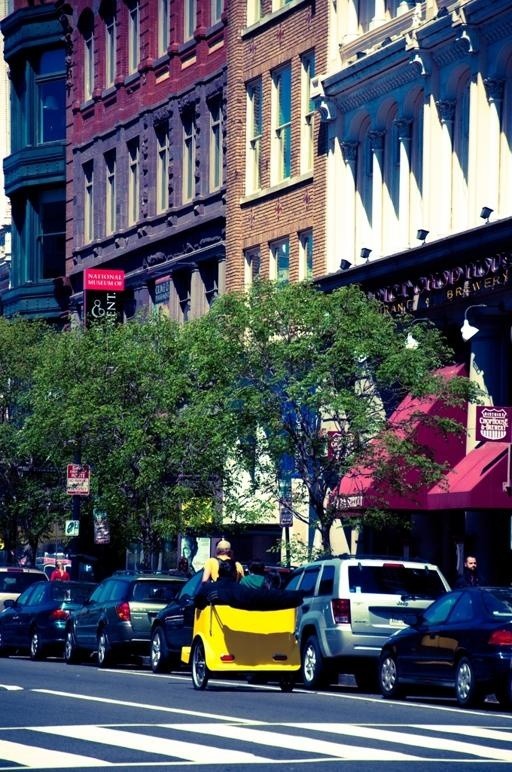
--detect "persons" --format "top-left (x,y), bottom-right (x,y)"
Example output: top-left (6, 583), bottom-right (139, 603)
top-left (454, 555), bottom-right (489, 588)
top-left (50, 560), bottom-right (70, 581)
top-left (173, 558), bottom-right (192, 578)
top-left (201, 540), bottom-right (272, 591)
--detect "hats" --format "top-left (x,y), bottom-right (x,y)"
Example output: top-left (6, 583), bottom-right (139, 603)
top-left (216, 540), bottom-right (231, 551)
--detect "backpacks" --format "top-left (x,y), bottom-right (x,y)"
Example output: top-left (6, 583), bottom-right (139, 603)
top-left (214, 556), bottom-right (237, 584)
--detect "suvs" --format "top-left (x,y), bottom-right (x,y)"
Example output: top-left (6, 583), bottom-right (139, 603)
top-left (247, 552), bottom-right (456, 693)
top-left (149, 563), bottom-right (299, 675)
top-left (63, 570), bottom-right (190, 669)
top-left (0, 566), bottom-right (50, 611)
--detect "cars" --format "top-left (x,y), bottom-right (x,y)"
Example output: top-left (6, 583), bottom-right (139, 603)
top-left (376, 587), bottom-right (512, 709)
top-left (0, 581), bottom-right (102, 662)
